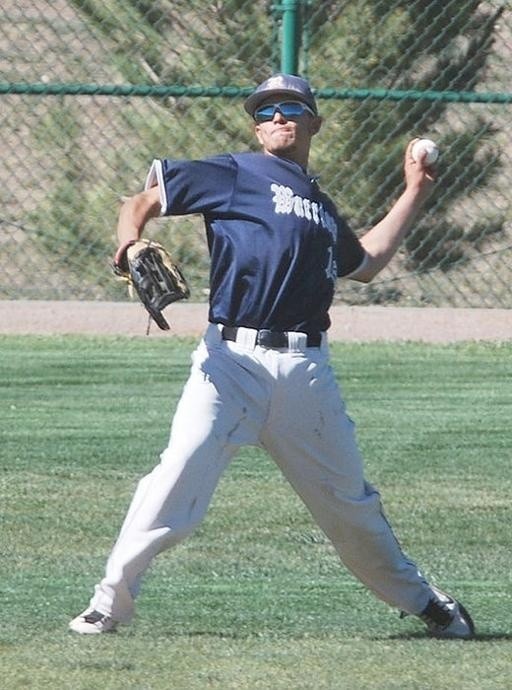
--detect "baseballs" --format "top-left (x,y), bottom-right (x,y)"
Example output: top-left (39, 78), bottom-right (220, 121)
top-left (411, 139), bottom-right (440, 166)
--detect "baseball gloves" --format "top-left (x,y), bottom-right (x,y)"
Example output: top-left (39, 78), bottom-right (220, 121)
top-left (129, 239), bottom-right (190, 332)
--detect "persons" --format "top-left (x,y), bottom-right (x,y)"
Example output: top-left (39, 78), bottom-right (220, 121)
top-left (67, 71), bottom-right (477, 642)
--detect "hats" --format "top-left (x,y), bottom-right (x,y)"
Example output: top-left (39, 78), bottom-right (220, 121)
top-left (244, 73), bottom-right (317, 118)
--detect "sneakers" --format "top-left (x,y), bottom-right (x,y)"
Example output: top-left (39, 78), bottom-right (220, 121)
top-left (414, 581), bottom-right (474, 640)
top-left (68, 605), bottom-right (118, 635)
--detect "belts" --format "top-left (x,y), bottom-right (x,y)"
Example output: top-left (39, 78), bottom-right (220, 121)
top-left (221, 326), bottom-right (321, 347)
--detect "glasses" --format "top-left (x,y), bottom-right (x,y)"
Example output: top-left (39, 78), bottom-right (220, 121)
top-left (251, 101), bottom-right (315, 123)
